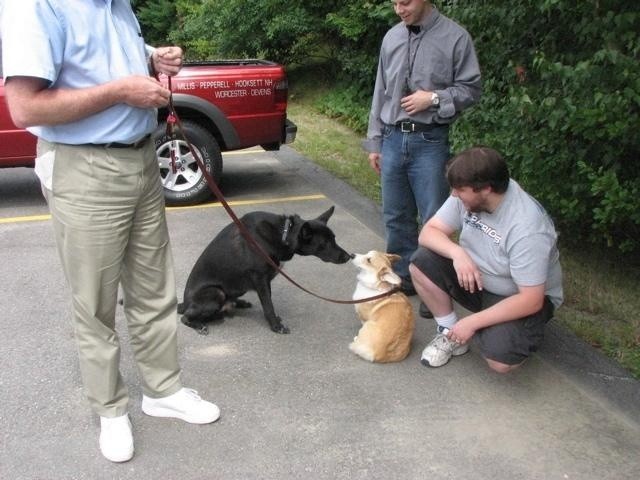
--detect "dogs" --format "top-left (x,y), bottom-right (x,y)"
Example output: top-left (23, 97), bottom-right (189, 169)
top-left (117, 199), bottom-right (350, 339)
top-left (345, 249), bottom-right (415, 365)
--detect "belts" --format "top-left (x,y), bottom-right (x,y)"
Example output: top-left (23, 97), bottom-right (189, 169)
top-left (88, 133), bottom-right (151, 150)
top-left (392, 121), bottom-right (447, 133)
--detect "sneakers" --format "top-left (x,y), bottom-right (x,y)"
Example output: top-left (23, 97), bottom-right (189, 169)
top-left (141, 386), bottom-right (220, 424)
top-left (100, 411), bottom-right (134, 462)
top-left (421, 325), bottom-right (469, 368)
top-left (400, 286), bottom-right (434, 318)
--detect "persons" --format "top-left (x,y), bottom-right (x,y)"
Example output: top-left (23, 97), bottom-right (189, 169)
top-left (407, 144), bottom-right (564, 374)
top-left (366, 1), bottom-right (485, 321)
top-left (0, 0), bottom-right (220, 463)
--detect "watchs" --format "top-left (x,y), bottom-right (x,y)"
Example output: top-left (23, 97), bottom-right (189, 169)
top-left (430, 91), bottom-right (440, 108)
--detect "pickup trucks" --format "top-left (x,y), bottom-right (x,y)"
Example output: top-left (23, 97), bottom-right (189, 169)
top-left (0, 38), bottom-right (296, 208)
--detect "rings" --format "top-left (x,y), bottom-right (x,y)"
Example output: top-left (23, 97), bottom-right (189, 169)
top-left (455, 339), bottom-right (460, 345)
